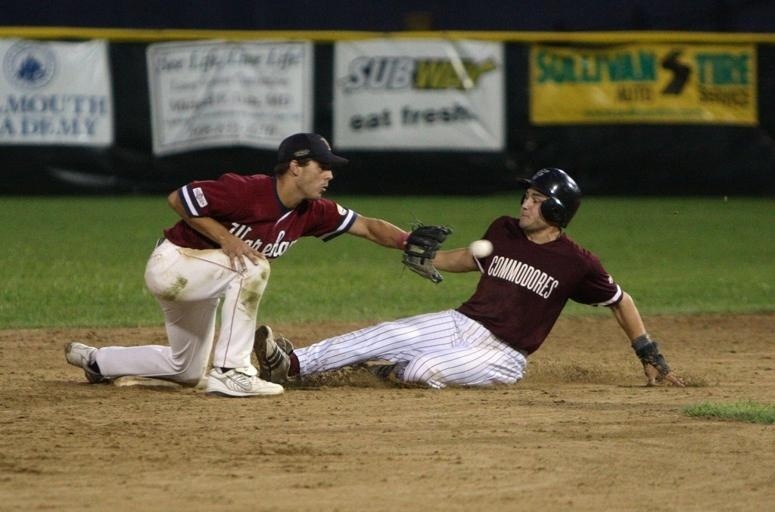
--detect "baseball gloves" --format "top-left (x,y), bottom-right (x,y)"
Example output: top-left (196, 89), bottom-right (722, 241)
top-left (403, 220), bottom-right (453, 283)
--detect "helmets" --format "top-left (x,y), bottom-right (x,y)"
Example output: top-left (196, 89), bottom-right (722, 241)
top-left (515, 167), bottom-right (583, 230)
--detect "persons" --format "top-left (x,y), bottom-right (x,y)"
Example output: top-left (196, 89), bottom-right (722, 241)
top-left (63, 131), bottom-right (452, 400)
top-left (253, 163), bottom-right (687, 389)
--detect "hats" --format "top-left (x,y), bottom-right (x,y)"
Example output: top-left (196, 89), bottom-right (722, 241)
top-left (276, 132), bottom-right (350, 170)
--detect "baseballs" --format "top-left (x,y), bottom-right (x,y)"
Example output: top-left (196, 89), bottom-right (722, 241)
top-left (468, 239), bottom-right (493, 258)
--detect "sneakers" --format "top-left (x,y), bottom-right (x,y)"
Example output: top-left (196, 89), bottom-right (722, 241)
top-left (254, 324), bottom-right (295, 383)
top-left (204, 365), bottom-right (287, 399)
top-left (361, 360), bottom-right (400, 388)
top-left (65, 341), bottom-right (112, 385)
